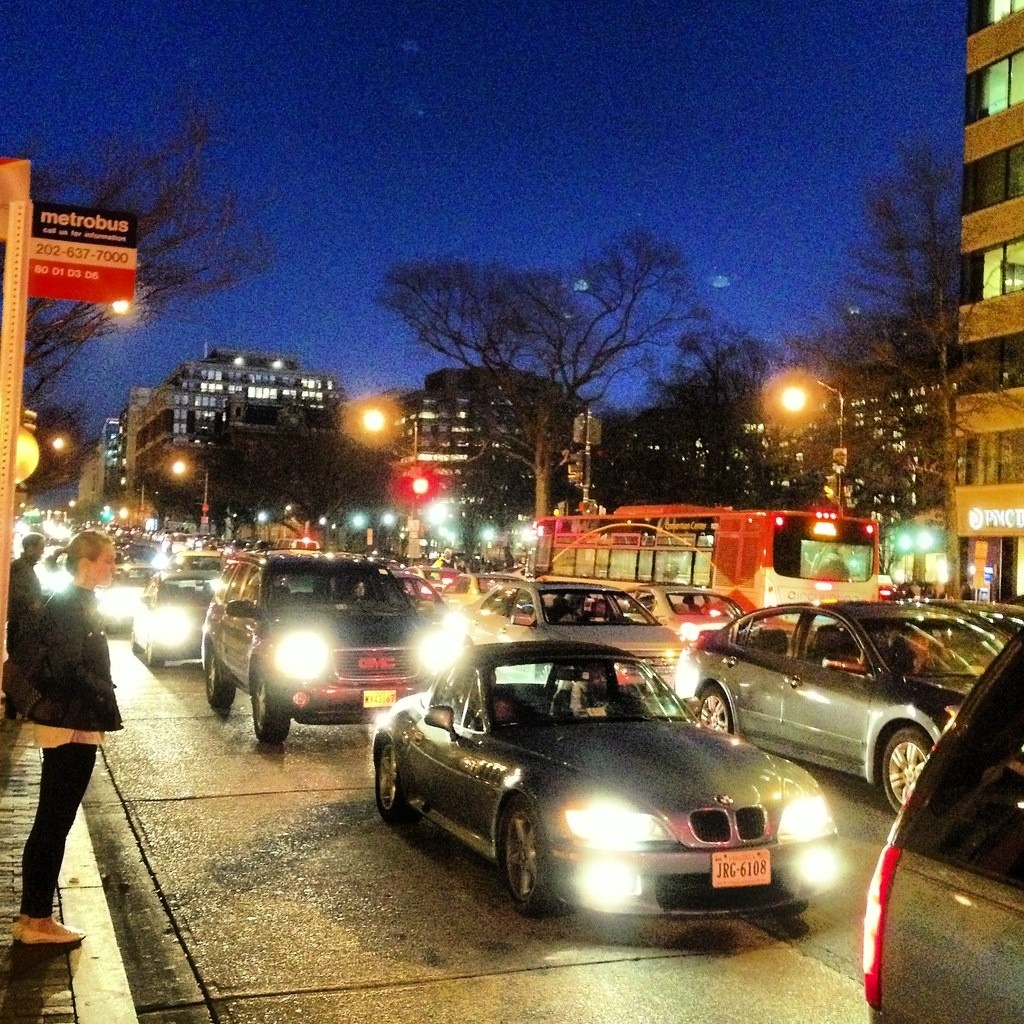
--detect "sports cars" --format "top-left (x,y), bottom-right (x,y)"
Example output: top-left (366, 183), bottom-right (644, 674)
top-left (369, 638), bottom-right (850, 919)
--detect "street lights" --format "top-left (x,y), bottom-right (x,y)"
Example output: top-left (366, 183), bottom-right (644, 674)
top-left (353, 401), bottom-right (419, 516)
top-left (172, 459), bottom-right (209, 529)
top-left (776, 375), bottom-right (842, 513)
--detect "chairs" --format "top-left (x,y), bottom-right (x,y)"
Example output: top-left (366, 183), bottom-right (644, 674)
top-left (748, 625), bottom-right (789, 654)
top-left (581, 597), bottom-right (596, 619)
top-left (549, 669), bottom-right (583, 718)
top-left (808, 623), bottom-right (842, 666)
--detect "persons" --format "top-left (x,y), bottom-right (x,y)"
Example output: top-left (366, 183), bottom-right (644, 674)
top-left (467, 664), bottom-right (642, 735)
top-left (683, 595), bottom-right (701, 614)
top-left (10, 529), bottom-right (126, 945)
top-left (341, 573), bottom-right (377, 605)
top-left (5, 532), bottom-right (47, 658)
top-left (835, 628), bottom-right (934, 677)
top-left (441, 548), bottom-right (457, 570)
top-left (540, 590), bottom-right (622, 627)
top-left (893, 576), bottom-right (975, 602)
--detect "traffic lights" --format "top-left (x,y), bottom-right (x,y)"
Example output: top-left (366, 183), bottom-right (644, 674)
top-left (411, 477), bottom-right (428, 494)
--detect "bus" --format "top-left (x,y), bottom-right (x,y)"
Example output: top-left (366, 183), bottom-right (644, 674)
top-left (522, 505), bottom-right (880, 614)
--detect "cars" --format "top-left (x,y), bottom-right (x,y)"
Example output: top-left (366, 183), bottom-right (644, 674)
top-left (441, 574), bottom-right (533, 598)
top-left (457, 581), bottom-right (688, 688)
top-left (671, 603), bottom-right (1017, 817)
top-left (856, 625), bottom-right (1024, 1024)
top-left (616, 585), bottom-right (746, 647)
top-left (32, 517), bottom-right (408, 668)
top-left (400, 565), bottom-right (464, 582)
top-left (392, 573), bottom-right (474, 672)
top-left (902, 586), bottom-right (1024, 628)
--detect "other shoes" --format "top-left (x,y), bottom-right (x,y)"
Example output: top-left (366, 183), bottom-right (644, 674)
top-left (20, 924), bottom-right (87, 944)
top-left (10, 921), bottom-right (26, 940)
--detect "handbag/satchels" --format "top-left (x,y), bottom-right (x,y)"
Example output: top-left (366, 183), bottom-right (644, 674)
top-left (1, 656), bottom-right (41, 718)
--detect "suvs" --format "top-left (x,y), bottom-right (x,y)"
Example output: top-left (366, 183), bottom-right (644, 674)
top-left (201, 549), bottom-right (437, 742)
